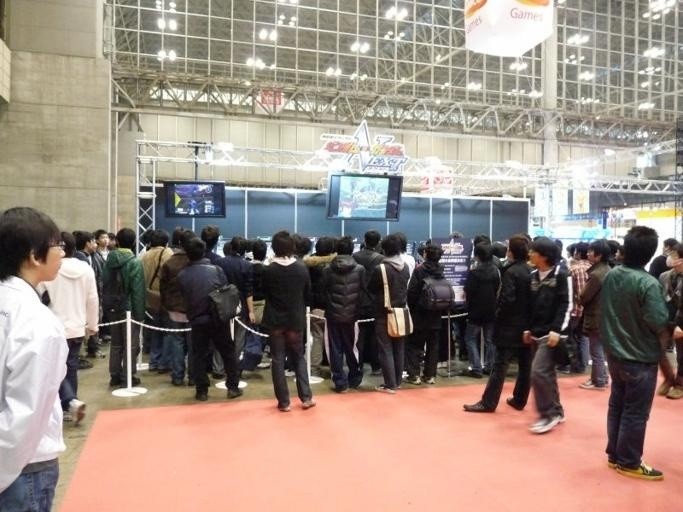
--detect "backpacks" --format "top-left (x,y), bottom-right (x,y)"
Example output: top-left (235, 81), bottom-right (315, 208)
top-left (417, 272), bottom-right (454, 312)
top-left (207, 265), bottom-right (239, 326)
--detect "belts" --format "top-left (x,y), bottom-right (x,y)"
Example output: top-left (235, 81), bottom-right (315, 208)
top-left (21, 457), bottom-right (58, 474)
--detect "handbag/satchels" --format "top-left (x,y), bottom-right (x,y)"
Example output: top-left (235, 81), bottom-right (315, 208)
top-left (386, 307), bottom-right (414, 338)
top-left (144, 288), bottom-right (161, 314)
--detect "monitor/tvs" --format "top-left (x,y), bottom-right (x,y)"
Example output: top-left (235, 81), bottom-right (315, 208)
top-left (326, 172), bottom-right (403, 222)
top-left (163, 180), bottom-right (227, 218)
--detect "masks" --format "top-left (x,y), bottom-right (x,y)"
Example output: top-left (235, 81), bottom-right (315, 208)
top-left (665, 255), bottom-right (682, 268)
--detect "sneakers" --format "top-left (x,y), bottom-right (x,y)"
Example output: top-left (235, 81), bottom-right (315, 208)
top-left (68, 398), bottom-right (85, 425)
top-left (528, 413), bottom-right (567, 434)
top-left (77, 335), bottom-right (139, 388)
top-left (279, 405), bottom-right (290, 411)
top-left (608, 458), bottom-right (617, 468)
top-left (284, 369), bottom-right (296, 376)
top-left (302, 399), bottom-right (315, 408)
top-left (63, 410), bottom-right (72, 421)
top-left (615, 457), bottom-right (663, 480)
top-left (311, 352), bottom-right (491, 393)
top-left (554, 361), bottom-right (608, 390)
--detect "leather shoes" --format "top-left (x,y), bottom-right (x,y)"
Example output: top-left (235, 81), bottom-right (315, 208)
top-left (506, 396), bottom-right (522, 410)
top-left (665, 386), bottom-right (682, 399)
top-left (463, 400), bottom-right (494, 412)
top-left (148, 343), bottom-right (258, 401)
top-left (658, 374), bottom-right (675, 395)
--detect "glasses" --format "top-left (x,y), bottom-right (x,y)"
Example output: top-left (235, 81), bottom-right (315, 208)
top-left (49, 240), bottom-right (66, 251)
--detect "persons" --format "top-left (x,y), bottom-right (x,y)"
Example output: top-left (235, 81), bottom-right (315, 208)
top-left (648, 239), bottom-right (683, 400)
top-left (597, 226), bottom-right (671, 481)
top-left (43, 226), bottom-right (447, 425)
top-left (0, 207), bottom-right (69, 512)
top-left (464, 235), bottom-right (623, 435)
